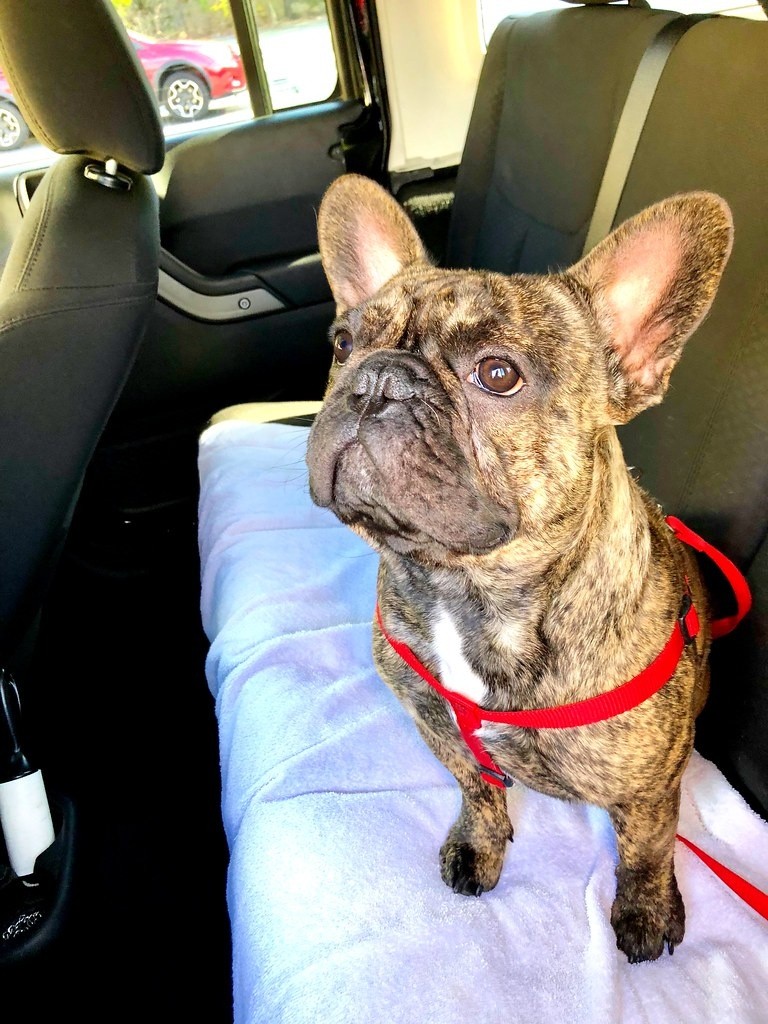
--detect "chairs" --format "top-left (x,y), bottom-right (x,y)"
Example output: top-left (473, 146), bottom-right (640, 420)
top-left (3, 0), bottom-right (240, 583)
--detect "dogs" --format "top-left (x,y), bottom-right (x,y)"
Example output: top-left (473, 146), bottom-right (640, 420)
top-left (303, 173), bottom-right (733, 968)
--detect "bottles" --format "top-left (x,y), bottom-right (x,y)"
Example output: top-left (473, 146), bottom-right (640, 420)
top-left (0, 670), bottom-right (55, 886)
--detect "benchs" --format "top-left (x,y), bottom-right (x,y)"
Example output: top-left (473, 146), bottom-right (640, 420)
top-left (194, 0), bottom-right (768, 1022)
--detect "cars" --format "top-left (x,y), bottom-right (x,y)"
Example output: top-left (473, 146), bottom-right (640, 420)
top-left (1, 25), bottom-right (251, 153)
